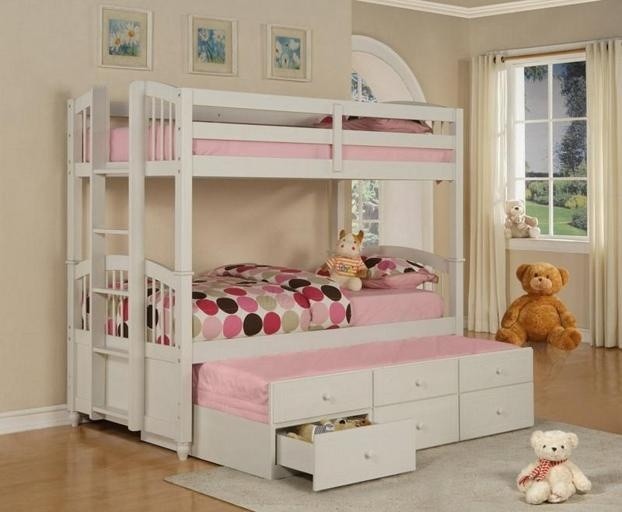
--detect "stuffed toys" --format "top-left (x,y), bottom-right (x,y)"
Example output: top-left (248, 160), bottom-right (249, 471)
top-left (286, 417), bottom-right (372, 444)
top-left (321, 230), bottom-right (372, 293)
top-left (497, 262), bottom-right (582, 350)
top-left (515, 429), bottom-right (592, 504)
top-left (504, 200), bottom-right (541, 238)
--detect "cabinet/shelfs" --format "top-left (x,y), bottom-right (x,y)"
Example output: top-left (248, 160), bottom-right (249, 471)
top-left (140, 334), bottom-right (535, 492)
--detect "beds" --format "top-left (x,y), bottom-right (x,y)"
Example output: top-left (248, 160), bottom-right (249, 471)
top-left (66, 80), bottom-right (464, 462)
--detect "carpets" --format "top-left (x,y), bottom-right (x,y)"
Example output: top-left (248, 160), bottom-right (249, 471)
top-left (163, 419), bottom-right (622, 511)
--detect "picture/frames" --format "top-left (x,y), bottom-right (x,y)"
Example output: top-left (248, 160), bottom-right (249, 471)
top-left (264, 24), bottom-right (312, 82)
top-left (97, 4), bottom-right (154, 71)
top-left (186, 14), bottom-right (238, 78)
